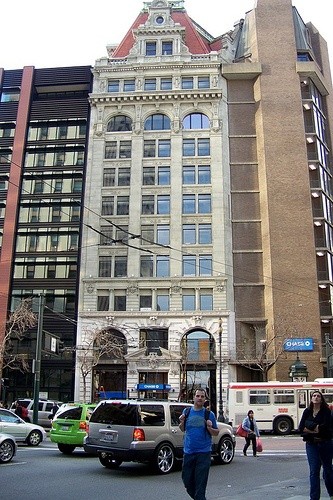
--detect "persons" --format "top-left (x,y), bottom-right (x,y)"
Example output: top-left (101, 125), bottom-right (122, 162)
top-left (180, 388), bottom-right (219, 500)
top-left (15, 401), bottom-right (28, 422)
top-left (47, 403), bottom-right (59, 420)
top-left (242, 410), bottom-right (260, 457)
top-left (299, 391), bottom-right (333, 500)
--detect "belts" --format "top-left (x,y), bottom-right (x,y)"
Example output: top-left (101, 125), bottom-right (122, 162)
top-left (314, 440), bottom-right (322, 442)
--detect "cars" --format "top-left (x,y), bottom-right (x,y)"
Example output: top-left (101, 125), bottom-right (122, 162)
top-left (0, 432), bottom-right (18, 464)
top-left (0, 407), bottom-right (47, 447)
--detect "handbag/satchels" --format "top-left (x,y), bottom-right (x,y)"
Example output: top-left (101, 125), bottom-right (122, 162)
top-left (236, 426), bottom-right (248, 437)
top-left (248, 432), bottom-right (256, 439)
top-left (256, 437), bottom-right (262, 452)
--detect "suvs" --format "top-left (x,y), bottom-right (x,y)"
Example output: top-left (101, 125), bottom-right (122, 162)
top-left (9, 397), bottom-right (64, 428)
top-left (49, 402), bottom-right (98, 454)
top-left (83, 400), bottom-right (236, 475)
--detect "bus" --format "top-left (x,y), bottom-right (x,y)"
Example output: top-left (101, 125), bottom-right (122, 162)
top-left (227, 377), bottom-right (333, 436)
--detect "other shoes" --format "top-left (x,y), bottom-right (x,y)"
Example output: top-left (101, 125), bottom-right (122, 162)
top-left (254, 455), bottom-right (259, 457)
top-left (243, 450), bottom-right (247, 456)
